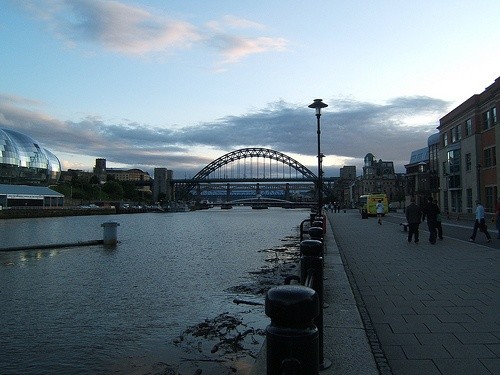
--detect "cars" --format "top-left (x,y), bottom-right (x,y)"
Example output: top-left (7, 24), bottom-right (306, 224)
top-left (121, 204), bottom-right (163, 208)
top-left (76, 204), bottom-right (99, 208)
top-left (199, 200), bottom-right (213, 204)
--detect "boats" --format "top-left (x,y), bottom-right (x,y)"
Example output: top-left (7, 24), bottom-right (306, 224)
top-left (167, 207), bottom-right (191, 212)
top-left (252, 204), bottom-right (269, 209)
top-left (221, 205), bottom-right (232, 209)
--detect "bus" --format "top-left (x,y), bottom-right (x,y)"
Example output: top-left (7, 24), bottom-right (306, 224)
top-left (359, 192), bottom-right (389, 217)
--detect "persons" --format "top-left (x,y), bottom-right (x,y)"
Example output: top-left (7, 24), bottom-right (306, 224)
top-left (495, 198), bottom-right (500, 239)
top-left (376, 200), bottom-right (384, 225)
top-left (328, 201), bottom-right (340, 213)
top-left (432, 200), bottom-right (443, 240)
top-left (422, 197), bottom-right (437, 245)
top-left (405, 202), bottom-right (421, 244)
top-left (389, 202), bottom-right (392, 212)
top-left (468, 200), bottom-right (492, 242)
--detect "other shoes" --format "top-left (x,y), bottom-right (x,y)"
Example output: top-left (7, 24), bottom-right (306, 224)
top-left (496, 237), bottom-right (500, 239)
top-left (409, 242), bottom-right (411, 243)
top-left (479, 225), bottom-right (487, 232)
top-left (416, 242), bottom-right (418, 244)
top-left (469, 239), bottom-right (475, 241)
top-left (487, 239), bottom-right (491, 242)
top-left (378, 222), bottom-right (382, 225)
top-left (437, 237), bottom-right (441, 239)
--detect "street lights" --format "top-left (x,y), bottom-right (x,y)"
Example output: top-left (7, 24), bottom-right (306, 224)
top-left (309, 99), bottom-right (328, 215)
